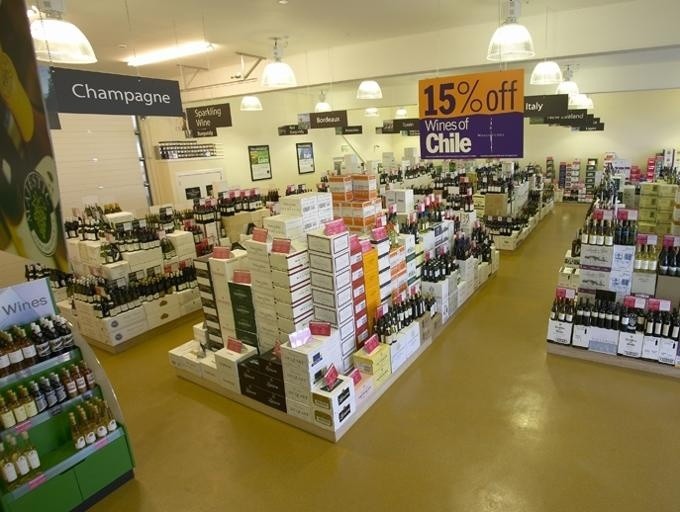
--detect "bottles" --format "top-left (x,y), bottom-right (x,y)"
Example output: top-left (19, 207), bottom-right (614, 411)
top-left (658, 165), bottom-right (680, 185)
top-left (0, 314), bottom-right (119, 492)
top-left (286, 176), bottom-right (330, 196)
top-left (372, 293), bottom-right (438, 345)
top-left (215, 190), bottom-right (278, 217)
top-left (570, 165), bottom-right (637, 258)
top-left (379, 161), bottom-right (552, 283)
top-left (549, 294), bottom-right (680, 340)
top-left (24, 198), bottom-right (227, 319)
top-left (635, 242), bottom-right (680, 278)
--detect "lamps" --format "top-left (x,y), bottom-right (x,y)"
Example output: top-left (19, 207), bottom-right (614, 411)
top-left (486, 0), bottom-right (593, 111)
top-left (239, 94), bottom-right (263, 111)
top-left (29, 0), bottom-right (98, 65)
top-left (315, 79), bottom-right (407, 120)
top-left (259, 35), bottom-right (295, 86)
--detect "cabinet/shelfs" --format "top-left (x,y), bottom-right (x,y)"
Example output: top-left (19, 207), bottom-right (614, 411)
top-left (0, 277), bottom-right (136, 512)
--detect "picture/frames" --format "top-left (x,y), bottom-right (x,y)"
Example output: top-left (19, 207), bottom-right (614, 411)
top-left (247, 145), bottom-right (272, 181)
top-left (296, 142), bottom-right (315, 174)
